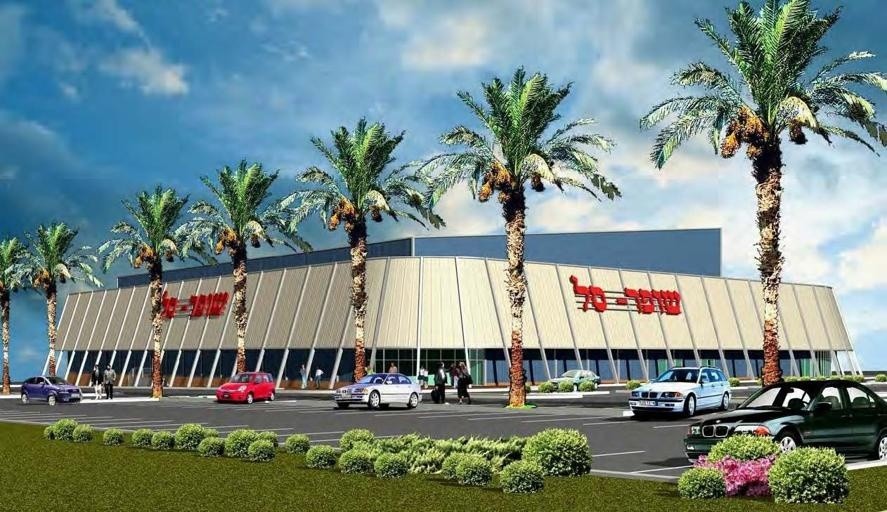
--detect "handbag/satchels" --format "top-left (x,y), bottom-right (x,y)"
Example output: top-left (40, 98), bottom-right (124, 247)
top-left (431, 390), bottom-right (440, 403)
top-left (468, 375), bottom-right (473, 384)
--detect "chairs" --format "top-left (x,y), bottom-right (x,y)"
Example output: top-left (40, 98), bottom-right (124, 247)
top-left (853, 397), bottom-right (870, 410)
top-left (824, 395), bottom-right (839, 411)
top-left (788, 398), bottom-right (804, 409)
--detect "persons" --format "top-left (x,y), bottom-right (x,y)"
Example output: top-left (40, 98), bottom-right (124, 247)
top-left (365, 361), bottom-right (473, 405)
top-left (300, 364), bottom-right (307, 387)
top-left (315, 367), bottom-right (324, 389)
top-left (103, 364), bottom-right (116, 399)
top-left (91, 363), bottom-right (103, 399)
